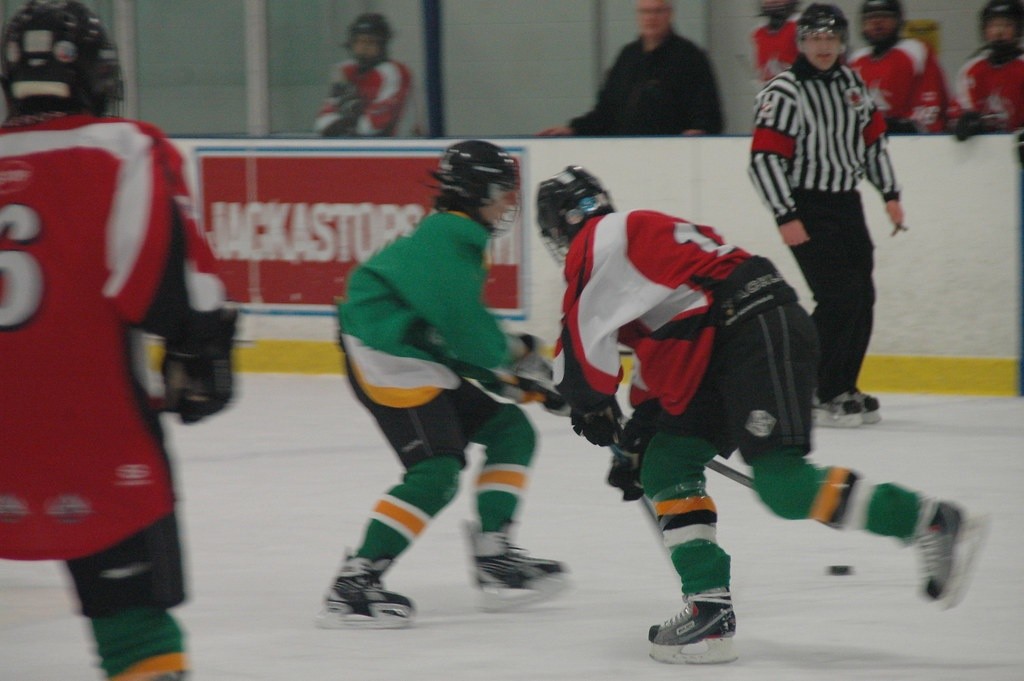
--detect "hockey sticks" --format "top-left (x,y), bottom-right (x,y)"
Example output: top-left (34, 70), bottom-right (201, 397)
top-left (535, 381), bottom-right (758, 491)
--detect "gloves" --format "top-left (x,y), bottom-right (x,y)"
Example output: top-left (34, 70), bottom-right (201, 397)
top-left (956, 111), bottom-right (989, 141)
top-left (881, 116), bottom-right (919, 137)
top-left (501, 330), bottom-right (553, 403)
top-left (609, 424), bottom-right (652, 503)
top-left (163, 304), bottom-right (238, 423)
top-left (549, 366), bottom-right (622, 447)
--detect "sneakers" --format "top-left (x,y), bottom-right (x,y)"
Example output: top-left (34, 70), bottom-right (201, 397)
top-left (464, 517), bottom-right (573, 611)
top-left (908, 490), bottom-right (984, 610)
top-left (809, 384), bottom-right (862, 427)
top-left (648, 586), bottom-right (738, 665)
top-left (318, 547), bottom-right (414, 628)
top-left (847, 389), bottom-right (882, 423)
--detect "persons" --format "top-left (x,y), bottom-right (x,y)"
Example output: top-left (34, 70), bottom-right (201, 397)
top-left (942, 0), bottom-right (1024, 165)
top-left (0, 0), bottom-right (241, 681)
top-left (323, 139), bottom-right (568, 616)
top-left (749, 5), bottom-right (905, 414)
top-left (534, 164), bottom-right (963, 646)
top-left (749, 0), bottom-right (804, 97)
top-left (845, 0), bottom-right (948, 137)
top-left (311, 11), bottom-right (411, 137)
top-left (536, 0), bottom-right (726, 137)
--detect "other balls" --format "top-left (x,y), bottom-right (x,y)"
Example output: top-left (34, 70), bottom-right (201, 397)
top-left (828, 565), bottom-right (851, 575)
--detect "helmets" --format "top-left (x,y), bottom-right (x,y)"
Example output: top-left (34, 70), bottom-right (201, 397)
top-left (861, 0), bottom-right (905, 44)
top-left (759, 0), bottom-right (797, 17)
top-left (796, 5), bottom-right (848, 55)
top-left (979, 0), bottom-right (1024, 52)
top-left (428, 140), bottom-right (519, 236)
top-left (0, 0), bottom-right (125, 121)
top-left (537, 165), bottom-right (616, 264)
top-left (348, 13), bottom-right (390, 62)
